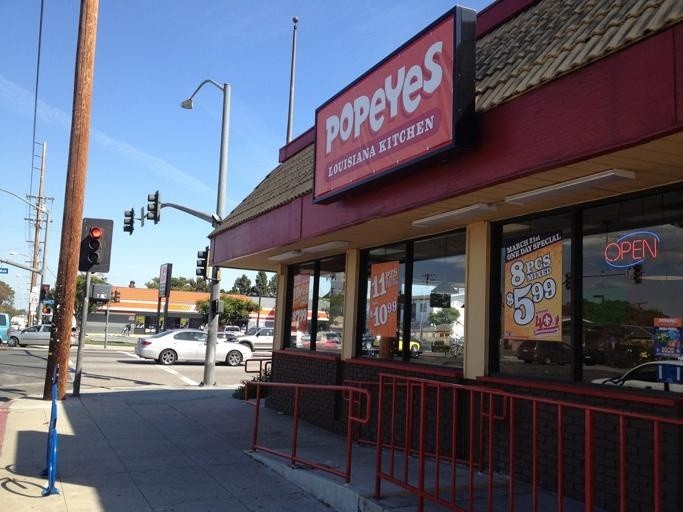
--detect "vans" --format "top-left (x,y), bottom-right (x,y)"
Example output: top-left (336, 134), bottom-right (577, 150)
top-left (0, 312), bottom-right (11, 345)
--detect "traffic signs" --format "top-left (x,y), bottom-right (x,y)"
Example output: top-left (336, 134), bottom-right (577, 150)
top-left (0, 268), bottom-right (8, 274)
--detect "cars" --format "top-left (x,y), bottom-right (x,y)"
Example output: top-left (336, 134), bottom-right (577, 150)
top-left (134, 329), bottom-right (252, 367)
top-left (517, 340), bottom-right (572, 365)
top-left (217, 326), bottom-right (420, 356)
top-left (8, 324), bottom-right (79, 347)
top-left (592, 360), bottom-right (683, 393)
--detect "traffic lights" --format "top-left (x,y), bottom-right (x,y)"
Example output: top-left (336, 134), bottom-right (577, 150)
top-left (123, 209), bottom-right (134, 234)
top-left (196, 246), bottom-right (210, 279)
top-left (147, 191), bottom-right (160, 224)
top-left (80, 218), bottom-right (113, 272)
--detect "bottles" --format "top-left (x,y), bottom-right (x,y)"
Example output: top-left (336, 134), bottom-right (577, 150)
top-left (379, 336), bottom-right (395, 361)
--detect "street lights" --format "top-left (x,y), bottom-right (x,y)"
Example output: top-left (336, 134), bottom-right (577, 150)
top-left (183, 79), bottom-right (232, 386)
top-left (11, 251), bottom-right (32, 323)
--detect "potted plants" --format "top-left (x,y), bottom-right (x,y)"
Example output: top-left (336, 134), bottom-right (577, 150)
top-left (429, 307), bottom-right (460, 337)
